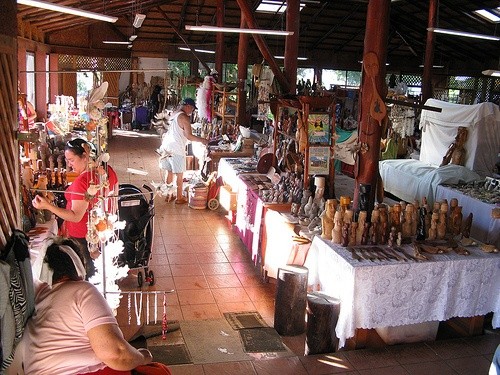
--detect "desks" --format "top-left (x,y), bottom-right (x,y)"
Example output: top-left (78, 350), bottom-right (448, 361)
top-left (378, 158), bottom-right (500, 247)
top-left (217, 158), bottom-right (500, 350)
top-left (190, 123), bottom-right (254, 173)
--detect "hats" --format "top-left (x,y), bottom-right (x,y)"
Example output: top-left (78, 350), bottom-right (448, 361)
top-left (181, 98), bottom-right (198, 110)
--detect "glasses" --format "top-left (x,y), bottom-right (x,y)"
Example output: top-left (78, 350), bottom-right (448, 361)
top-left (66, 140), bottom-right (83, 151)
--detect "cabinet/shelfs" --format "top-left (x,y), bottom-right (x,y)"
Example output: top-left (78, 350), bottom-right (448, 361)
top-left (274, 103), bottom-right (336, 199)
top-left (213, 90), bottom-right (239, 125)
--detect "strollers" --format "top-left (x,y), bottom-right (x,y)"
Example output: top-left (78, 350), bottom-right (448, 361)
top-left (116, 182), bottom-right (156, 288)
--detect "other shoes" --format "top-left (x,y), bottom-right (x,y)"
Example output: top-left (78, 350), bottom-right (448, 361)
top-left (165, 195), bottom-right (176, 202)
top-left (175, 196), bottom-right (188, 204)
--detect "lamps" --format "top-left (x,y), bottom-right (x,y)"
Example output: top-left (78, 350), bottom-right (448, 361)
top-left (133, 0), bottom-right (146, 28)
top-left (16, 0), bottom-right (118, 23)
top-left (185, 0), bottom-right (294, 36)
top-left (427, 0), bottom-right (500, 41)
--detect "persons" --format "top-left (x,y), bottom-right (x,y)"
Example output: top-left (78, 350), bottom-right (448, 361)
top-left (27, 101), bottom-right (37, 125)
top-left (150, 84), bottom-right (162, 119)
top-left (320, 195), bottom-right (463, 247)
top-left (141, 82), bottom-right (150, 101)
top-left (160, 97), bottom-right (210, 204)
top-left (32, 139), bottom-right (119, 285)
top-left (8, 237), bottom-right (171, 375)
